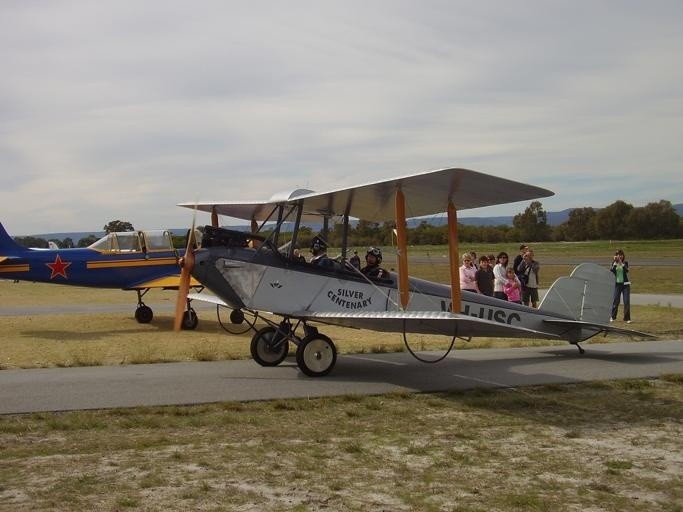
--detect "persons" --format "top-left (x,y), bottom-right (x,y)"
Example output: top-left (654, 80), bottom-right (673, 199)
top-left (459, 245), bottom-right (539, 308)
top-left (294, 249), bottom-right (306, 264)
top-left (360, 247), bottom-right (389, 281)
top-left (608, 248), bottom-right (632, 324)
top-left (349, 249), bottom-right (360, 270)
top-left (310, 233), bottom-right (330, 266)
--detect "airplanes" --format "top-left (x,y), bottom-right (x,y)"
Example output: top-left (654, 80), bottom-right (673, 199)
top-left (0, 219), bottom-right (259, 325)
top-left (172, 169), bottom-right (658, 375)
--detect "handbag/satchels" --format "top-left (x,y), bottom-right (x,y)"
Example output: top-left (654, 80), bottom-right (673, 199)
top-left (518, 274), bottom-right (528, 287)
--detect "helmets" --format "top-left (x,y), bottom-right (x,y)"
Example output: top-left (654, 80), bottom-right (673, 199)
top-left (366, 247), bottom-right (382, 264)
top-left (311, 234), bottom-right (328, 253)
